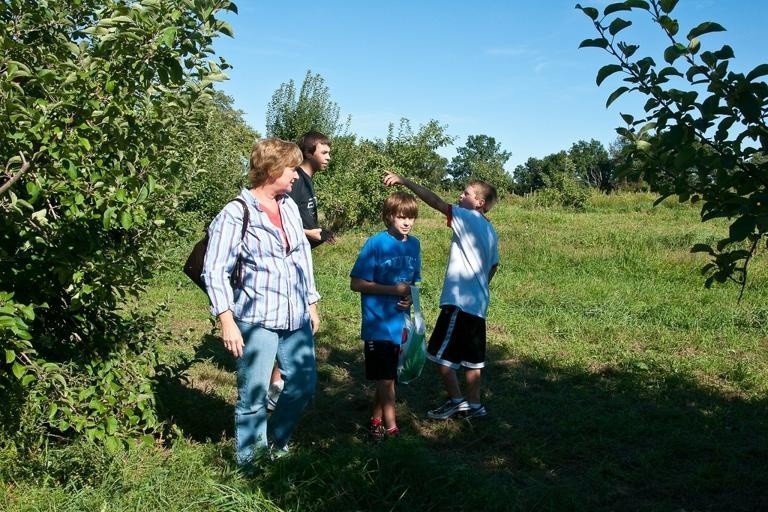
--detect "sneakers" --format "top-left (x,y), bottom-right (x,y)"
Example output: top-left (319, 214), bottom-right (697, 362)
top-left (366, 417), bottom-right (385, 440)
top-left (453, 402), bottom-right (489, 422)
top-left (268, 381), bottom-right (286, 412)
top-left (386, 427), bottom-right (406, 442)
top-left (234, 440), bottom-right (290, 475)
top-left (425, 396), bottom-right (471, 421)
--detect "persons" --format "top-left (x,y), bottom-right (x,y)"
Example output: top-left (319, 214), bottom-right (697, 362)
top-left (200, 136), bottom-right (324, 473)
top-left (348, 190), bottom-right (425, 443)
top-left (289, 129), bottom-right (332, 253)
top-left (379, 167), bottom-right (506, 423)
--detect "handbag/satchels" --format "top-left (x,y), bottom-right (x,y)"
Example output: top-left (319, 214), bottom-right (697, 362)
top-left (185, 234), bottom-right (237, 304)
top-left (395, 312), bottom-right (428, 386)
